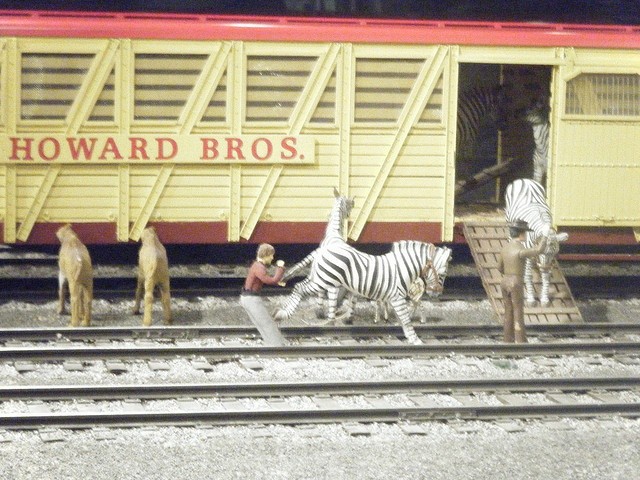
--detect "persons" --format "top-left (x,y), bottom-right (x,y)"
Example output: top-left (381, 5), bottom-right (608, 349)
top-left (238, 243), bottom-right (289, 341)
top-left (497, 219), bottom-right (549, 344)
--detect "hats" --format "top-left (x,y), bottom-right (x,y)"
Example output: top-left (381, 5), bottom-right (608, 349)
top-left (505, 218), bottom-right (535, 233)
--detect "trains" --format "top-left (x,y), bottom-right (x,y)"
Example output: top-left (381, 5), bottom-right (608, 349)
top-left (1, 10), bottom-right (640, 262)
